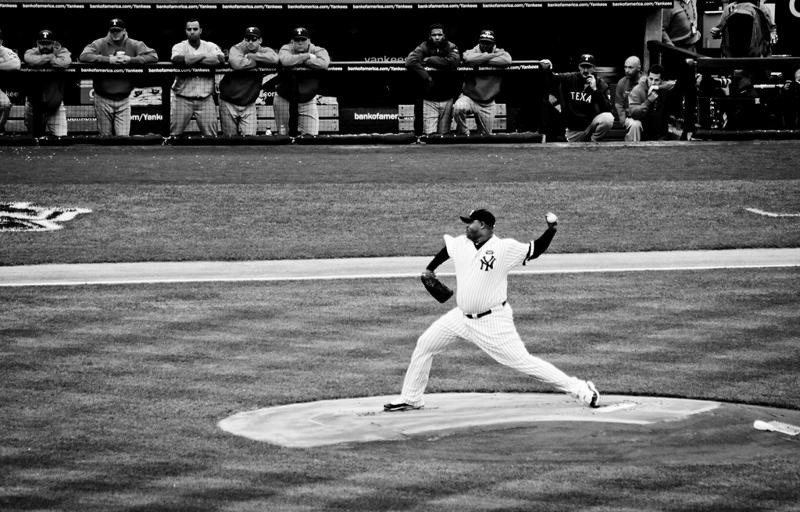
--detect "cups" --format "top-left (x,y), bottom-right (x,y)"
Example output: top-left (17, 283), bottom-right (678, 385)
top-left (115, 51), bottom-right (128, 59)
top-left (713, 28), bottom-right (720, 39)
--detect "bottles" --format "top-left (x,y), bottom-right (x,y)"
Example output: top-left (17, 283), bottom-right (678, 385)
top-left (264, 125), bottom-right (272, 133)
top-left (276, 124), bottom-right (288, 136)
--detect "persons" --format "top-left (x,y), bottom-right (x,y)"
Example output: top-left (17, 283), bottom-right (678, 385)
top-left (169, 18), bottom-right (225, 137)
top-left (23, 29), bottom-right (72, 137)
top-left (78, 18), bottom-right (159, 136)
top-left (451, 29), bottom-right (513, 136)
top-left (272, 27), bottom-right (331, 136)
top-left (0, 44), bottom-right (21, 136)
top-left (537, 1), bottom-right (800, 143)
top-left (383, 209), bottom-right (601, 410)
top-left (404, 23), bottom-right (461, 136)
top-left (219, 26), bottom-right (279, 137)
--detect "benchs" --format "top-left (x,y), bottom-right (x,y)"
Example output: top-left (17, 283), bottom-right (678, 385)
top-left (4, 105), bottom-right (97, 135)
top-left (184, 95), bottom-right (341, 132)
top-left (398, 104), bottom-right (507, 133)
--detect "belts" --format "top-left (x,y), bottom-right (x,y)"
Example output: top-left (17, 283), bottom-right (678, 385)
top-left (462, 299), bottom-right (508, 321)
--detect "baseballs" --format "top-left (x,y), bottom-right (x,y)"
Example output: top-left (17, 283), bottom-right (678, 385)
top-left (546, 212), bottom-right (557, 223)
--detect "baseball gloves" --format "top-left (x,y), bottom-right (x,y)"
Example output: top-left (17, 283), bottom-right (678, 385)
top-left (421, 275), bottom-right (454, 303)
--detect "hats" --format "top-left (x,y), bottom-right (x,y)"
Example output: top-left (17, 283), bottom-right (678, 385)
top-left (38, 30), bottom-right (54, 44)
top-left (108, 18), bottom-right (125, 33)
top-left (478, 29), bottom-right (495, 43)
top-left (579, 54), bottom-right (596, 68)
top-left (460, 209), bottom-right (495, 227)
top-left (292, 27), bottom-right (310, 40)
top-left (241, 26), bottom-right (262, 38)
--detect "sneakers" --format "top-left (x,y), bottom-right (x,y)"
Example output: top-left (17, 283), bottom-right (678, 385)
top-left (587, 379), bottom-right (600, 409)
top-left (382, 399), bottom-right (425, 412)
